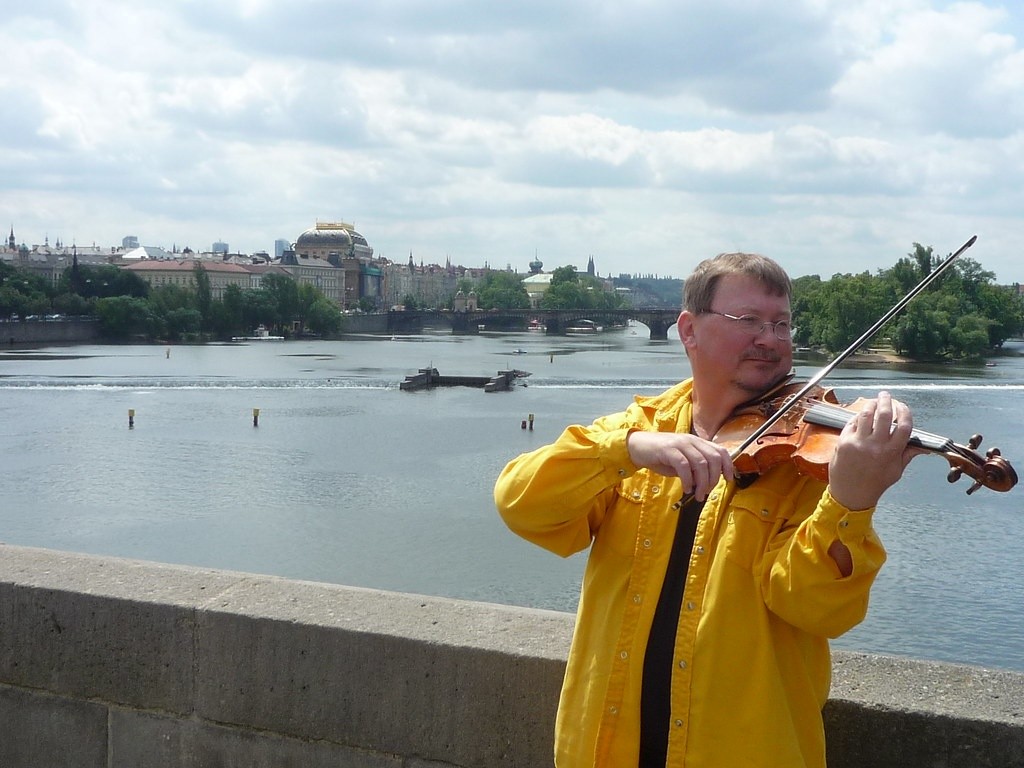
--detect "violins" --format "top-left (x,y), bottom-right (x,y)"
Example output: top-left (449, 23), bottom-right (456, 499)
top-left (711, 377), bottom-right (1020, 501)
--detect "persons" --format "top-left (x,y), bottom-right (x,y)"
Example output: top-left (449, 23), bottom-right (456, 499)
top-left (495, 252), bottom-right (930, 768)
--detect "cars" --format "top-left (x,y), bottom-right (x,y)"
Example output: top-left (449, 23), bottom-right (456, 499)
top-left (26, 314), bottom-right (63, 321)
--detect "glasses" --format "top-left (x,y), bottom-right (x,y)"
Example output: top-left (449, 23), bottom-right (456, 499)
top-left (700, 309), bottom-right (799, 340)
